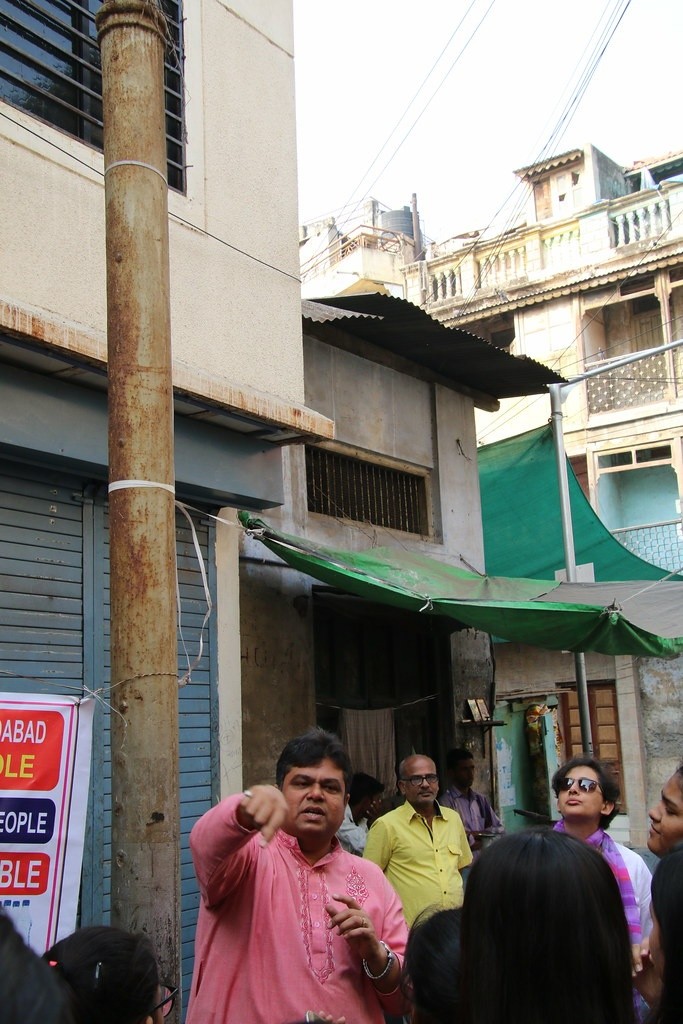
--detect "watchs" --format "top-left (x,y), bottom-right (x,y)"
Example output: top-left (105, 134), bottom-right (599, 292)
top-left (363, 941), bottom-right (395, 981)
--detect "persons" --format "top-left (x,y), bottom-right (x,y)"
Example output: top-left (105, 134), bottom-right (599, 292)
top-left (0, 903), bottom-right (180, 1024)
top-left (185, 725), bottom-right (683, 1024)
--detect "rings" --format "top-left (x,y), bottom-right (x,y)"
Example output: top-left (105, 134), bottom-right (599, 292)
top-left (362, 917), bottom-right (367, 928)
top-left (244, 790), bottom-right (253, 798)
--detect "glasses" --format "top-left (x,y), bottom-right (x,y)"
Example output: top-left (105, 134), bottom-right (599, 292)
top-left (557, 778), bottom-right (606, 793)
top-left (402, 775), bottom-right (438, 786)
top-left (136, 984), bottom-right (179, 1024)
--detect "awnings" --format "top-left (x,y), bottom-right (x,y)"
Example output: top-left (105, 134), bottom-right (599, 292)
top-left (235, 420), bottom-right (683, 661)
